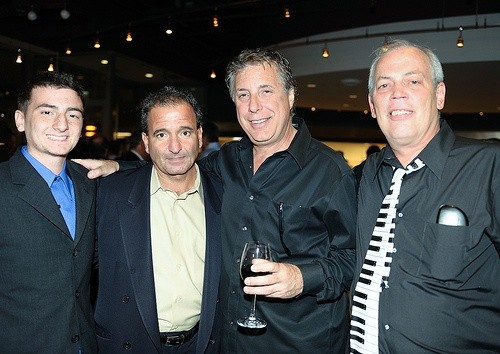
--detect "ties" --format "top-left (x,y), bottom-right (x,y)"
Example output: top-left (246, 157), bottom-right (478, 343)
top-left (349, 158), bottom-right (424, 354)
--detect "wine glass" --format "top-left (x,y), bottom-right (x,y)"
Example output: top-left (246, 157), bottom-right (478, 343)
top-left (236, 242), bottom-right (271, 328)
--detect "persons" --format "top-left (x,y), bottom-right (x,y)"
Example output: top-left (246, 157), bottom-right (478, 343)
top-left (114, 124), bottom-right (151, 162)
top-left (350, 40), bottom-right (500, 354)
top-left (94, 87), bottom-right (223, 354)
top-left (1, 74), bottom-right (98, 354)
top-left (71, 48), bottom-right (359, 354)
top-left (352, 144), bottom-right (381, 184)
top-left (196, 121), bottom-right (221, 160)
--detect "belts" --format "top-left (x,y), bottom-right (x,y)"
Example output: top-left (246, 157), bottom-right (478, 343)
top-left (160, 320), bottom-right (200, 347)
